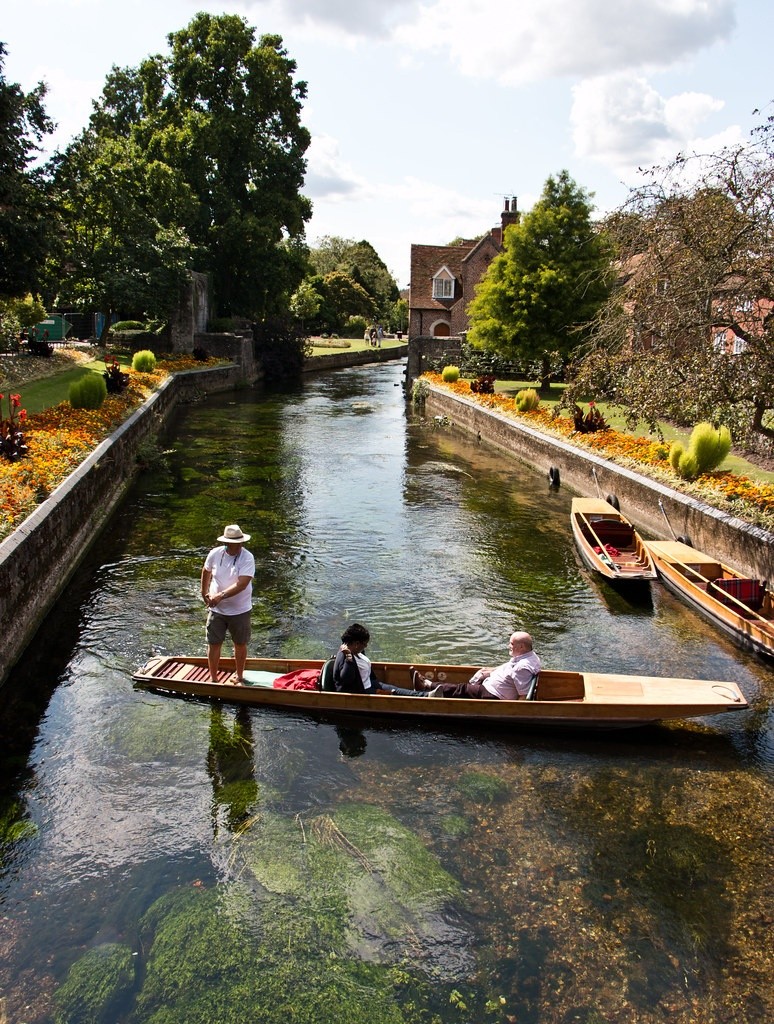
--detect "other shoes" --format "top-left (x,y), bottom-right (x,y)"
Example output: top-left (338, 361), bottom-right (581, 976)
top-left (409, 666), bottom-right (429, 692)
top-left (428, 684), bottom-right (444, 698)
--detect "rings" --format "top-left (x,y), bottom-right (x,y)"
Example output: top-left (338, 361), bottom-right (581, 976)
top-left (213, 605), bottom-right (215, 607)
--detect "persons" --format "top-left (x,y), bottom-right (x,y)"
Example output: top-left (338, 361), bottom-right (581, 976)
top-left (410, 632), bottom-right (541, 700)
top-left (20, 327), bottom-right (29, 344)
top-left (31, 325), bottom-right (39, 338)
top-left (333, 624), bottom-right (443, 698)
top-left (43, 328), bottom-right (49, 343)
top-left (201, 524), bottom-right (256, 686)
top-left (364, 325), bottom-right (384, 348)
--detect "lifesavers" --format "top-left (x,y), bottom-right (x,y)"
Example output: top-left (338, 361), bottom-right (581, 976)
top-left (676, 534), bottom-right (693, 547)
top-left (548, 464), bottom-right (561, 488)
top-left (606, 493), bottom-right (620, 508)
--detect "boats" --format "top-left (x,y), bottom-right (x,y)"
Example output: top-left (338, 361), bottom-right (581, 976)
top-left (131, 653), bottom-right (751, 721)
top-left (568, 494), bottom-right (659, 582)
top-left (643, 537), bottom-right (773, 660)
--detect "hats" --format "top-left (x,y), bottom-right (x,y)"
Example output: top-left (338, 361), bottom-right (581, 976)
top-left (216, 524), bottom-right (251, 544)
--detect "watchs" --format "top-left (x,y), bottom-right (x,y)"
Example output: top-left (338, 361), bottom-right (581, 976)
top-left (222, 592), bottom-right (228, 598)
